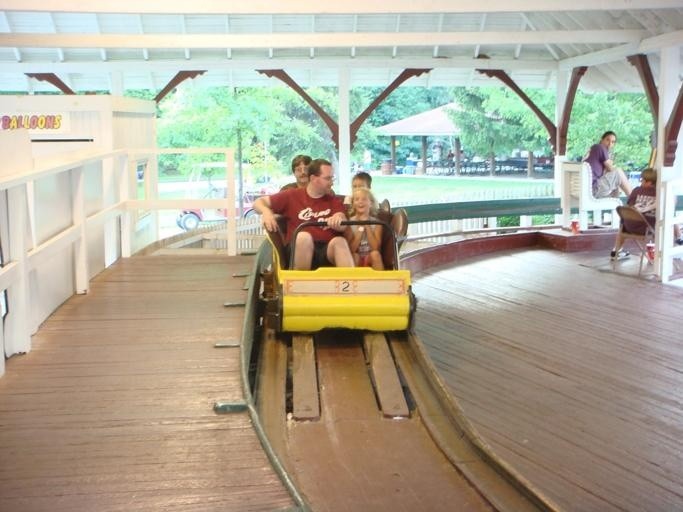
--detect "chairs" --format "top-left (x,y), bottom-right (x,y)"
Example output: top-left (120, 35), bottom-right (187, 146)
top-left (561, 161), bottom-right (622, 229)
top-left (614, 206), bottom-right (679, 276)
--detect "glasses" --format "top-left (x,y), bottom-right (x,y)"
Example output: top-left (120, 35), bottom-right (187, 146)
top-left (318, 173), bottom-right (337, 182)
top-left (292, 166), bottom-right (309, 174)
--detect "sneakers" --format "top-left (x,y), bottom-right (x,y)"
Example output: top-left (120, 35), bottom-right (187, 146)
top-left (673, 235), bottom-right (683, 247)
top-left (609, 246), bottom-right (630, 262)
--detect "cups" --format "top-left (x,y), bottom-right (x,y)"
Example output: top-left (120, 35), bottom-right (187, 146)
top-left (646, 244), bottom-right (654, 261)
top-left (571, 221), bottom-right (579, 232)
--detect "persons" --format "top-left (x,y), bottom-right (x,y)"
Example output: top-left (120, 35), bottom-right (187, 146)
top-left (345, 186), bottom-right (389, 270)
top-left (431, 138), bottom-right (443, 174)
top-left (579, 129), bottom-right (634, 199)
top-left (609, 167), bottom-right (683, 261)
top-left (446, 149), bottom-right (454, 175)
top-left (279, 153), bottom-right (335, 195)
top-left (251, 157), bottom-right (358, 270)
top-left (344, 171), bottom-right (388, 215)
top-left (459, 148), bottom-right (467, 174)
top-left (404, 151), bottom-right (417, 161)
